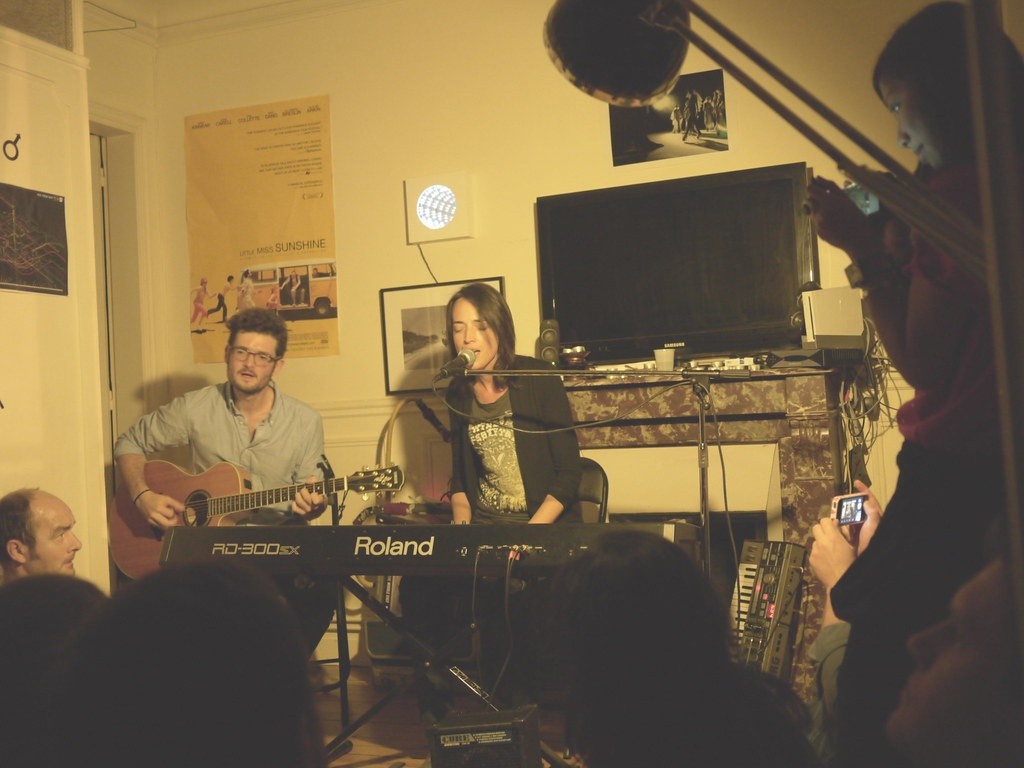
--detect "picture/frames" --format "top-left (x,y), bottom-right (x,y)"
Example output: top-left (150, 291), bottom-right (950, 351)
top-left (378, 276), bottom-right (505, 395)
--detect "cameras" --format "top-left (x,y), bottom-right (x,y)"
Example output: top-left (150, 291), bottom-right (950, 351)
top-left (840, 173), bottom-right (894, 223)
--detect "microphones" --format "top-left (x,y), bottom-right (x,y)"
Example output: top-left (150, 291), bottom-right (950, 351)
top-left (440, 350), bottom-right (474, 378)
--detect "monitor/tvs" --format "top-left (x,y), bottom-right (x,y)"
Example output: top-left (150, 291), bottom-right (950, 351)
top-left (536, 161), bottom-right (808, 363)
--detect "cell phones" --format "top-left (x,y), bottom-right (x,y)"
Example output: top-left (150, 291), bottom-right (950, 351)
top-left (829, 492), bottom-right (869, 526)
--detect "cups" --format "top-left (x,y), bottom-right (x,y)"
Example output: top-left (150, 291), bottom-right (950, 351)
top-left (654, 349), bottom-right (675, 373)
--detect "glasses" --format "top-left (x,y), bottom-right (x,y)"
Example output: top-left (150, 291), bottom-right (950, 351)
top-left (231, 344), bottom-right (280, 367)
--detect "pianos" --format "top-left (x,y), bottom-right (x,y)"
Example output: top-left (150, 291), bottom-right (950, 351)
top-left (725, 538), bottom-right (808, 680)
top-left (160, 522), bottom-right (704, 577)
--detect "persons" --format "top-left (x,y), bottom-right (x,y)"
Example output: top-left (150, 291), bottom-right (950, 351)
top-left (190, 277), bottom-right (217, 332)
top-left (206, 276), bottom-right (238, 323)
top-left (266, 286), bottom-right (279, 316)
top-left (238, 269), bottom-right (262, 309)
top-left (801, 0), bottom-right (1024, 723)
top-left (400, 283), bottom-right (584, 738)
top-left (537, 530), bottom-right (819, 767)
top-left (845, 504), bottom-right (851, 522)
top-left (50, 558), bottom-right (322, 768)
top-left (281, 268), bottom-right (305, 308)
top-left (0, 488), bottom-right (83, 587)
top-left (311, 268), bottom-right (321, 279)
top-left (2, 577), bottom-right (111, 730)
top-left (670, 87), bottom-right (724, 142)
top-left (115, 307), bottom-right (339, 670)
top-left (885, 550), bottom-right (1022, 768)
top-left (850, 503), bottom-right (856, 522)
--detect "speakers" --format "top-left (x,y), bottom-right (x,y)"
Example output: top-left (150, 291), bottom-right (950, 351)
top-left (429, 704), bottom-right (542, 768)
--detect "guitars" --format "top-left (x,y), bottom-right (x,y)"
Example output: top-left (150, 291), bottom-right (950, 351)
top-left (107, 460), bottom-right (404, 580)
